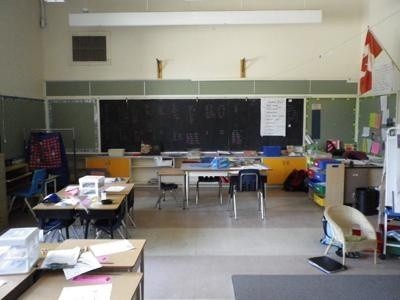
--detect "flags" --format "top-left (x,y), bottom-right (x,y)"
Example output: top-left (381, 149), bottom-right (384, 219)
top-left (359, 26), bottom-right (384, 94)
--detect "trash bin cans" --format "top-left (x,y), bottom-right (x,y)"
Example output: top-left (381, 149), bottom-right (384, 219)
top-left (355, 187), bottom-right (380, 216)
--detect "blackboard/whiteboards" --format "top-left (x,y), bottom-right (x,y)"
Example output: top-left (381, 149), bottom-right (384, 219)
top-left (98, 96), bottom-right (306, 155)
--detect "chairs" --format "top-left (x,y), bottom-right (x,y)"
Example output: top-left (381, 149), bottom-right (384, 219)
top-left (154, 157), bottom-right (273, 219)
top-left (324, 204), bottom-right (378, 267)
top-left (8, 167), bottom-right (137, 239)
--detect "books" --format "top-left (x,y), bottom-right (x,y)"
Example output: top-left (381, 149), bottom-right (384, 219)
top-left (307, 255), bottom-right (346, 275)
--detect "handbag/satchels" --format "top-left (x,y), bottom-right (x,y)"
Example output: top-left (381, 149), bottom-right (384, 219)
top-left (320, 216), bottom-right (340, 246)
top-left (284, 169), bottom-right (303, 191)
top-left (28, 135), bottom-right (63, 169)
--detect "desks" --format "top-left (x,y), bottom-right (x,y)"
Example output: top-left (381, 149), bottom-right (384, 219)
top-left (0, 238), bottom-right (146, 299)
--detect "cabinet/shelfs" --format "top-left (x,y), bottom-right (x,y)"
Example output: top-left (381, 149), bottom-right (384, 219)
top-left (87, 156), bottom-right (131, 190)
top-left (131, 157), bottom-right (175, 188)
top-left (307, 157), bottom-right (345, 206)
top-left (262, 156), bottom-right (307, 189)
